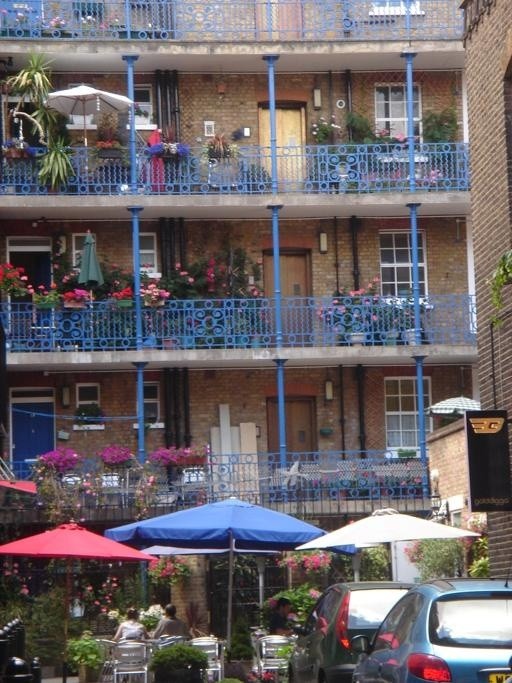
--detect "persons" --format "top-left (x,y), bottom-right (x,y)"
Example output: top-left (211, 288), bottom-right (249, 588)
top-left (269, 597), bottom-right (295, 637)
top-left (153, 603), bottom-right (193, 641)
top-left (113, 609), bottom-right (151, 641)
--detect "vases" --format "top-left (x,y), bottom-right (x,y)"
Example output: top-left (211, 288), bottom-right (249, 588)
top-left (319, 425), bottom-right (334, 437)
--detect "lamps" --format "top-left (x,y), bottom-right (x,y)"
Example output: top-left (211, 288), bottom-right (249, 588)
top-left (432, 485), bottom-right (450, 521)
top-left (323, 377), bottom-right (335, 402)
top-left (310, 82), bottom-right (324, 111)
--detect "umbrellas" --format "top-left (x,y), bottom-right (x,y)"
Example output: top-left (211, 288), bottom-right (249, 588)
top-left (294, 507), bottom-right (481, 582)
top-left (77, 229), bottom-right (104, 351)
top-left (1, 519), bottom-right (159, 683)
top-left (45, 83), bottom-right (136, 195)
top-left (424, 395), bottom-right (482, 421)
top-left (104, 495), bottom-right (360, 663)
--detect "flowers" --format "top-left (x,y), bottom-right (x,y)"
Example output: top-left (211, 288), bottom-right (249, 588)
top-left (1, 0), bottom-right (170, 38)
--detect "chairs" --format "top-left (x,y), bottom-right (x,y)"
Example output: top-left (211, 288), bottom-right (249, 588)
top-left (94, 626), bottom-right (295, 682)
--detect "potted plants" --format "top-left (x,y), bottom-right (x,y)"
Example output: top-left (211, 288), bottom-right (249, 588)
top-left (72, 400), bottom-right (107, 427)
top-left (63, 629), bottom-right (108, 683)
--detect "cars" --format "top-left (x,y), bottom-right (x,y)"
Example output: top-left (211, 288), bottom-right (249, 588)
top-left (351, 574), bottom-right (512, 682)
top-left (287, 581), bottom-right (413, 682)
